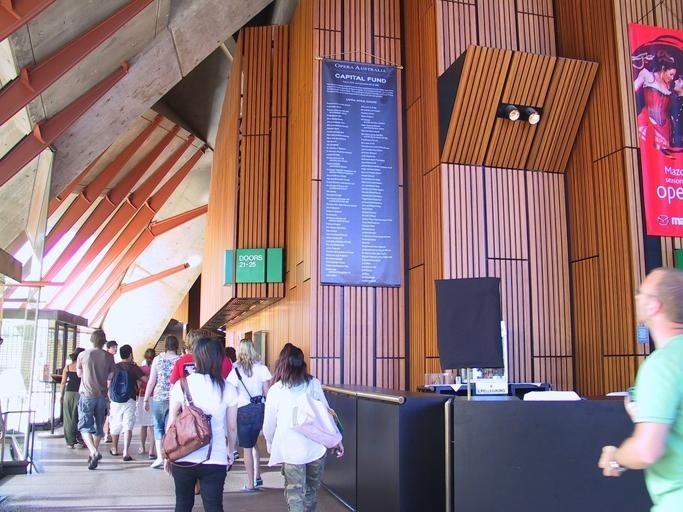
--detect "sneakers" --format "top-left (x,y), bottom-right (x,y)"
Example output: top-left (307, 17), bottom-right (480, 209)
top-left (103, 433), bottom-right (112, 444)
top-left (232, 450), bottom-right (240, 460)
top-left (151, 457), bottom-right (164, 468)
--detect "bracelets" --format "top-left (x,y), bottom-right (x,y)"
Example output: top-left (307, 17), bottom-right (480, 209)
top-left (163, 335), bottom-right (238, 512)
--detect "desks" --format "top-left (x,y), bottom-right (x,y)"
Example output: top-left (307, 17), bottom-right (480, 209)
top-left (443, 396), bottom-right (650, 512)
top-left (322, 377), bottom-right (445, 512)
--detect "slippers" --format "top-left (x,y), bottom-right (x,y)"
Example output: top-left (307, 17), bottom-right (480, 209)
top-left (148, 454), bottom-right (157, 460)
top-left (122, 456), bottom-right (133, 461)
top-left (241, 484), bottom-right (254, 493)
top-left (255, 479), bottom-right (264, 485)
top-left (110, 449), bottom-right (118, 456)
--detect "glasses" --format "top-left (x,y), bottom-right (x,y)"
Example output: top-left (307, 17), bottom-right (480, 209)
top-left (635, 289), bottom-right (657, 300)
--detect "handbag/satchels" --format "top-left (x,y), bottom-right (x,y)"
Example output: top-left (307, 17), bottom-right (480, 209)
top-left (161, 377), bottom-right (213, 468)
top-left (290, 377), bottom-right (342, 449)
top-left (234, 367), bottom-right (266, 404)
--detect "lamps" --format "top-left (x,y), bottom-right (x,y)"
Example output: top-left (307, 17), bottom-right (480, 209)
top-left (501, 103), bottom-right (520, 121)
top-left (524, 107), bottom-right (540, 124)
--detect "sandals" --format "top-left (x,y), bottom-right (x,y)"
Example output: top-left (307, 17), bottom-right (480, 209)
top-left (88, 455), bottom-right (95, 462)
top-left (88, 453), bottom-right (102, 469)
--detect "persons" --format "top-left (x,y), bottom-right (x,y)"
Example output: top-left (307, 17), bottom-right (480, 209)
top-left (633, 48), bottom-right (677, 150)
top-left (597, 267), bottom-right (683, 512)
top-left (143, 335), bottom-right (181, 468)
top-left (262, 342), bottom-right (345, 511)
top-left (76, 328), bottom-right (115, 470)
top-left (59, 347), bottom-right (87, 449)
top-left (169, 327), bottom-right (234, 392)
top-left (105, 340), bottom-right (118, 443)
top-left (225, 339), bottom-right (274, 493)
top-left (135, 348), bottom-right (157, 460)
top-left (668, 74), bottom-right (683, 148)
top-left (224, 347), bottom-right (237, 364)
top-left (109, 344), bottom-right (149, 461)
